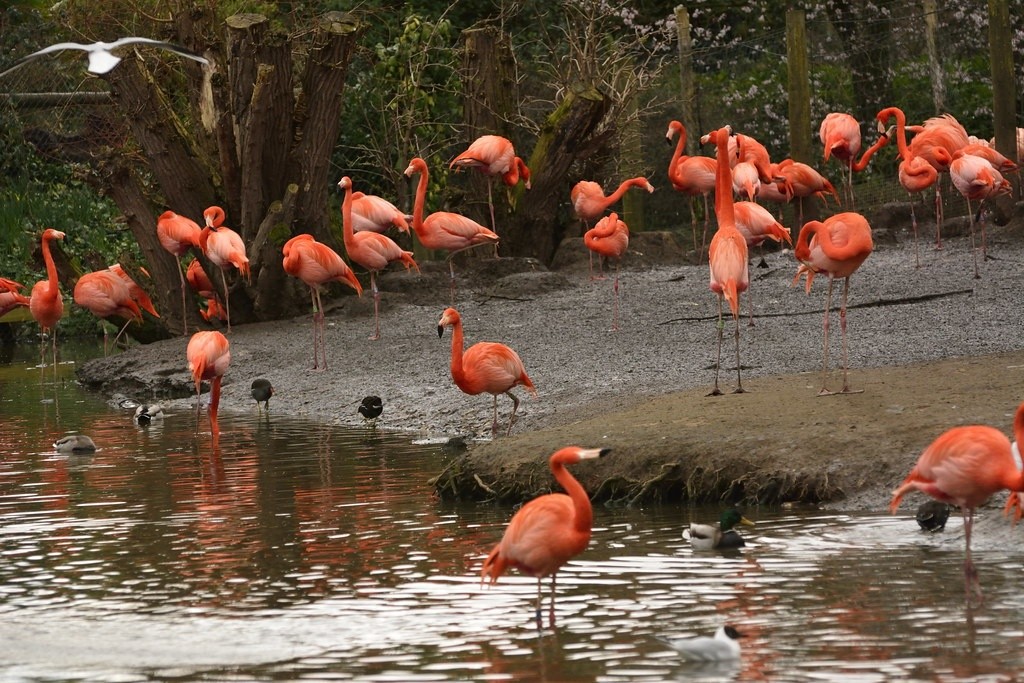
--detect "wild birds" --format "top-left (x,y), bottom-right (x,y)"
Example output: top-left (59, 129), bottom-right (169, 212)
top-left (657, 627), bottom-right (750, 664)
top-left (132, 405), bottom-right (165, 424)
top-left (687, 510), bottom-right (754, 553)
top-left (199, 206), bottom-right (252, 335)
top-left (0, 277), bottom-right (30, 318)
top-left (29, 229), bottom-right (68, 353)
top-left (357, 395), bottom-right (383, 423)
top-left (337, 157), bottom-right (502, 341)
top-left (283, 233), bottom-right (362, 370)
top-left (0, 35), bottom-right (211, 79)
top-left (156, 210), bottom-right (203, 333)
top-left (186, 256), bottom-right (227, 323)
top-left (52, 436), bottom-right (97, 453)
top-left (73, 264), bottom-right (161, 358)
top-left (450, 99), bottom-right (1024, 555)
top-left (480, 446), bottom-right (611, 616)
top-left (251, 378), bottom-right (275, 413)
top-left (914, 501), bottom-right (953, 534)
top-left (437, 306), bottom-right (537, 437)
top-left (187, 330), bottom-right (230, 438)
